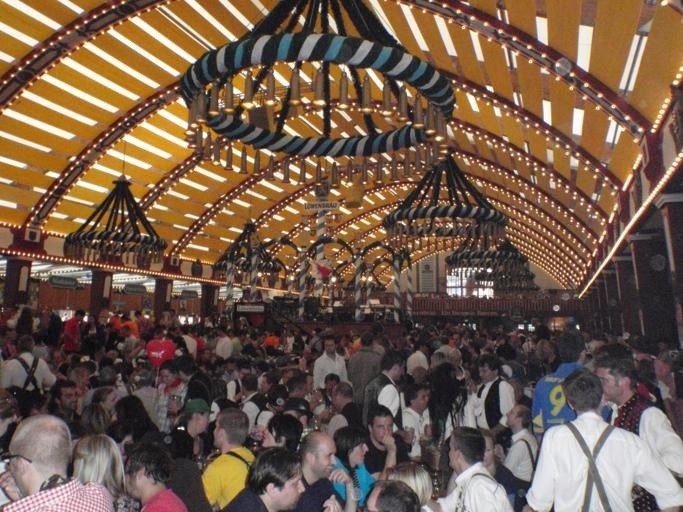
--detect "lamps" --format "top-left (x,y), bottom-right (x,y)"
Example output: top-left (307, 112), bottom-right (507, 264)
top-left (381, 148), bottom-right (508, 252)
top-left (445, 235), bottom-right (529, 281)
top-left (181, 0), bottom-right (457, 186)
top-left (492, 283), bottom-right (540, 295)
top-left (346, 261), bottom-right (388, 291)
top-left (475, 265), bottom-right (535, 289)
top-left (212, 196), bottom-right (283, 271)
top-left (63, 135), bottom-right (168, 267)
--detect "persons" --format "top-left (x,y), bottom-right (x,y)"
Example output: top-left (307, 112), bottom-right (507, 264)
top-left (657, 338), bottom-right (671, 360)
top-left (122, 444), bottom-right (188, 512)
top-left (445, 428), bottom-right (517, 507)
top-left (361, 351), bottom-right (413, 444)
top-left (266, 330), bottom-right (285, 356)
top-left (260, 374), bottom-right (277, 394)
top-left (199, 408), bottom-right (255, 509)
top-left (299, 372), bottom-right (314, 402)
top-left (476, 354), bottom-right (514, 447)
top-left (429, 366), bottom-right (480, 440)
top-left (73, 433), bottom-right (124, 496)
top-left (521, 368), bottom-right (683, 512)
top-left (241, 374), bottom-right (266, 430)
top-left (313, 336), bottom-right (352, 396)
top-left (596, 344), bottom-right (630, 359)
top-left (240, 317), bottom-right (248, 329)
top-left (215, 325), bottom-right (233, 362)
top-left (405, 341), bottom-right (429, 375)
top-left (330, 426), bottom-right (377, 507)
top-left (255, 385), bottom-right (287, 428)
top-left (285, 431), bottom-right (356, 512)
top-left (318, 382), bottom-right (362, 439)
top-left (286, 377), bottom-right (320, 413)
top-left (628, 334), bottom-right (653, 365)
top-left (401, 384), bottom-right (432, 460)
top-left (503, 404), bottom-right (537, 483)
top-left (411, 367), bottom-right (426, 384)
top-left (595, 356), bottom-right (683, 512)
top-left (347, 330), bottom-right (381, 406)
top-left (637, 359), bottom-right (678, 430)
top-left (261, 414), bottom-right (302, 453)
top-left (364, 404), bottom-right (411, 481)
top-left (0, 414), bottom-right (115, 512)
top-left (313, 374), bottom-right (339, 414)
top-left (432, 336), bottom-right (453, 361)
top-left (229, 328), bottom-right (242, 355)
top-left (285, 328), bottom-right (294, 352)
top-left (428, 426), bottom-right (516, 512)
top-left (0, 297), bottom-right (215, 448)
top-left (452, 334), bottom-right (460, 345)
top-left (251, 396), bottom-right (312, 441)
top-left (212, 357), bottom-right (226, 400)
top-left (293, 329), bottom-right (304, 356)
top-left (532, 331), bottom-right (609, 447)
top-left (366, 479), bottom-right (421, 512)
top-left (256, 361), bottom-right (268, 388)
top-left (219, 447), bottom-right (342, 512)
top-left (448, 347), bottom-right (471, 382)
top-left (336, 334), bottom-right (350, 360)
top-left (386, 461), bottom-right (442, 512)
top-left (227, 364), bottom-right (250, 403)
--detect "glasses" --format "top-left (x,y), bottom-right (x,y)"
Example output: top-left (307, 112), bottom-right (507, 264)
top-left (1, 452), bottom-right (31, 463)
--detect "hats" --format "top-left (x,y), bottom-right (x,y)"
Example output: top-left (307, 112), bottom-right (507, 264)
top-left (184, 399), bottom-right (215, 413)
top-left (281, 397), bottom-right (313, 415)
top-left (66, 355), bottom-right (96, 374)
